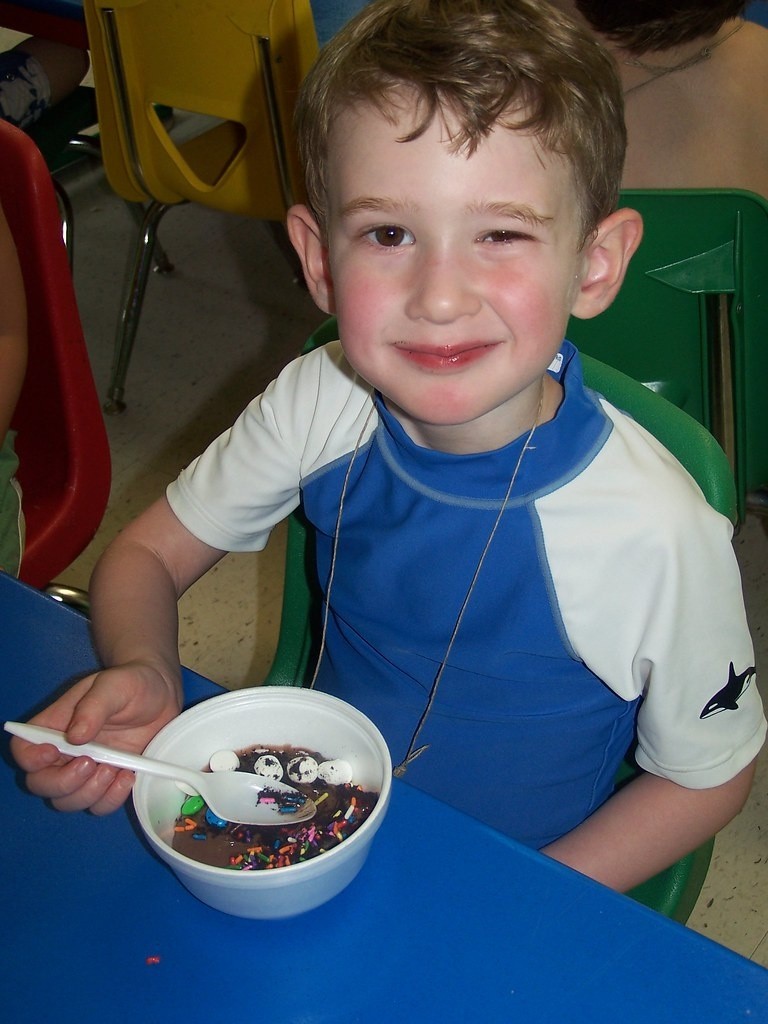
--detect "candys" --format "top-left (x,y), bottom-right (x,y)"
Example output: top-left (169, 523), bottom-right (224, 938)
top-left (254, 755), bottom-right (283, 781)
top-left (318, 760), bottom-right (351, 784)
top-left (210, 750), bottom-right (240, 771)
top-left (181, 796), bottom-right (204, 815)
top-left (206, 809), bottom-right (228, 828)
top-left (287, 757), bottom-right (318, 783)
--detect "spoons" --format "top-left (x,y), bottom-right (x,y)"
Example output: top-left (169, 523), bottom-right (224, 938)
top-left (2, 720), bottom-right (317, 827)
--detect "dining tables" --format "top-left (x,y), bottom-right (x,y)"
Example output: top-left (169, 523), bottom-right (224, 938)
top-left (1, 572), bottom-right (768, 1024)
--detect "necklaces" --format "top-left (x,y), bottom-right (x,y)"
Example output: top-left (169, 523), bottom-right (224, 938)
top-left (618, 24), bottom-right (743, 94)
top-left (309, 373), bottom-right (544, 779)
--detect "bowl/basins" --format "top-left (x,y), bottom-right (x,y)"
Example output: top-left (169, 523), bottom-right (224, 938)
top-left (132, 685), bottom-right (392, 921)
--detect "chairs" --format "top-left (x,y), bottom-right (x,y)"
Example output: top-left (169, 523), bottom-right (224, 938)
top-left (251, 309), bottom-right (744, 928)
top-left (552, 188), bottom-right (768, 528)
top-left (84, 1), bottom-right (367, 414)
top-left (0, 118), bottom-right (113, 590)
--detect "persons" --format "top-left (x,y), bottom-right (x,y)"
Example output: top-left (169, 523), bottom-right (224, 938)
top-left (574, 0), bottom-right (768, 204)
top-left (1, 34), bottom-right (91, 132)
top-left (7, 0), bottom-right (768, 898)
top-left (0, 211), bottom-right (31, 584)
